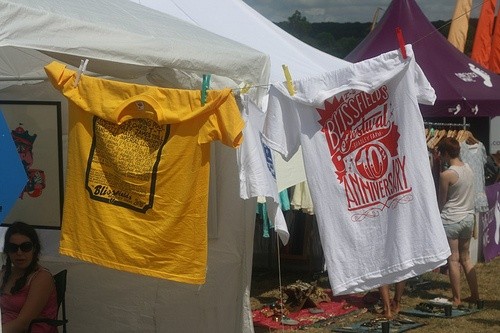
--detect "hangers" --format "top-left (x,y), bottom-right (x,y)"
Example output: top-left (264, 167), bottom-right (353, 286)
top-left (425, 122), bottom-right (479, 151)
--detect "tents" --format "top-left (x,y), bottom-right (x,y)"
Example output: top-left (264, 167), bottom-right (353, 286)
top-left (0, 0), bottom-right (271, 333)
top-left (341, 0), bottom-right (500, 130)
top-left (130, 0), bottom-right (353, 134)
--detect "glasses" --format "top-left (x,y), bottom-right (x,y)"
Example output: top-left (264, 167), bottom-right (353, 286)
top-left (6, 241), bottom-right (33, 253)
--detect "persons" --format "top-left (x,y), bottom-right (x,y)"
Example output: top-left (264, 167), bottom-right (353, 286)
top-left (376, 280), bottom-right (404, 318)
top-left (436, 137), bottom-right (480, 307)
top-left (0, 221), bottom-right (58, 333)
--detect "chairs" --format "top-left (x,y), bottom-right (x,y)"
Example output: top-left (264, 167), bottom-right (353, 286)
top-left (28, 269), bottom-right (68, 333)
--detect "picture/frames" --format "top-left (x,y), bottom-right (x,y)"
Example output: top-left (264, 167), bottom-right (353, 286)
top-left (0, 100), bottom-right (63, 230)
